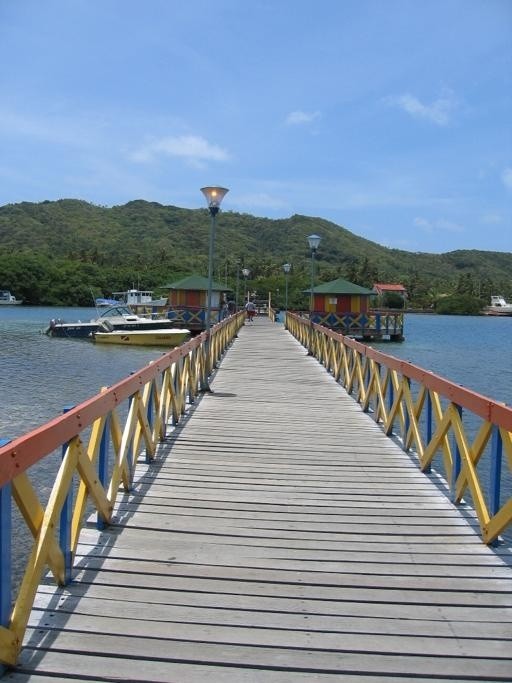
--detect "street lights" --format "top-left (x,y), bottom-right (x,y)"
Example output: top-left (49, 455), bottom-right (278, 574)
top-left (196, 181), bottom-right (231, 395)
top-left (281, 263), bottom-right (291, 312)
top-left (241, 267), bottom-right (251, 309)
top-left (305, 232), bottom-right (321, 357)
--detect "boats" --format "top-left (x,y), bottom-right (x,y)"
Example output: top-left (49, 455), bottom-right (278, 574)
top-left (95, 271), bottom-right (169, 308)
top-left (0, 289), bottom-right (24, 305)
top-left (485, 295), bottom-right (512, 317)
top-left (92, 319), bottom-right (193, 348)
top-left (42, 303), bottom-right (175, 339)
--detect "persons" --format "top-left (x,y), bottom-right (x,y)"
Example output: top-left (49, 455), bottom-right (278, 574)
top-left (244, 298), bottom-right (257, 322)
